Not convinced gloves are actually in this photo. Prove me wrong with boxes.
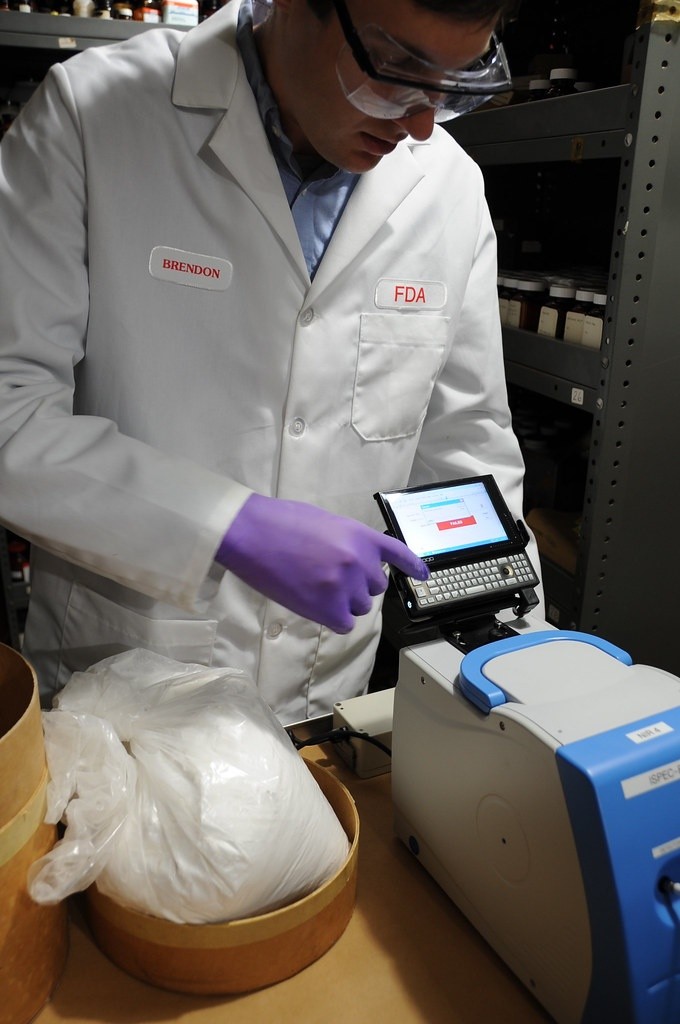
[213,491,429,635]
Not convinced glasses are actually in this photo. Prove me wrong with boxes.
[334,0,513,124]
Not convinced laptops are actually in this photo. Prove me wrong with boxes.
[374,474,539,617]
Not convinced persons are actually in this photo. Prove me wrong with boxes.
[1,0,548,728]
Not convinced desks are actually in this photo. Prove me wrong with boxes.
[34,739,554,1024]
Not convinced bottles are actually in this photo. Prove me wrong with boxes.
[545,69,578,99]
[498,267,608,350]
[523,80,550,104]
[513,407,570,516]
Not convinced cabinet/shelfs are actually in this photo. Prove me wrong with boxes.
[0,0,680,674]
